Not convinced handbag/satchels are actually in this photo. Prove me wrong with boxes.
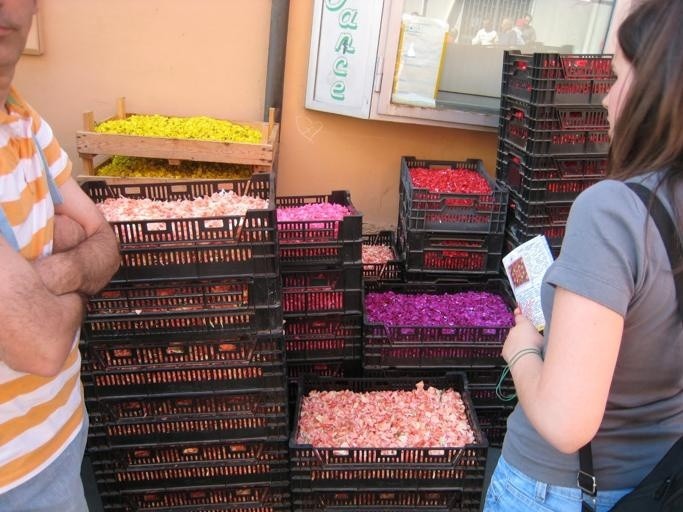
[547,181,682,511]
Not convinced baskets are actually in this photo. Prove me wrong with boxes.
[298,483,481,508]
[274,190,402,385]
[290,374,486,487]
[497,50,619,285]
[468,388,521,450]
[79,173,294,507]
[393,154,511,277]
[365,279,520,370]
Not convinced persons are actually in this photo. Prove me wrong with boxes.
[482,1,683,512]
[447,12,538,50]
[0,1,122,511]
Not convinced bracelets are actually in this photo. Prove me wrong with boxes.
[495,349,542,401]
